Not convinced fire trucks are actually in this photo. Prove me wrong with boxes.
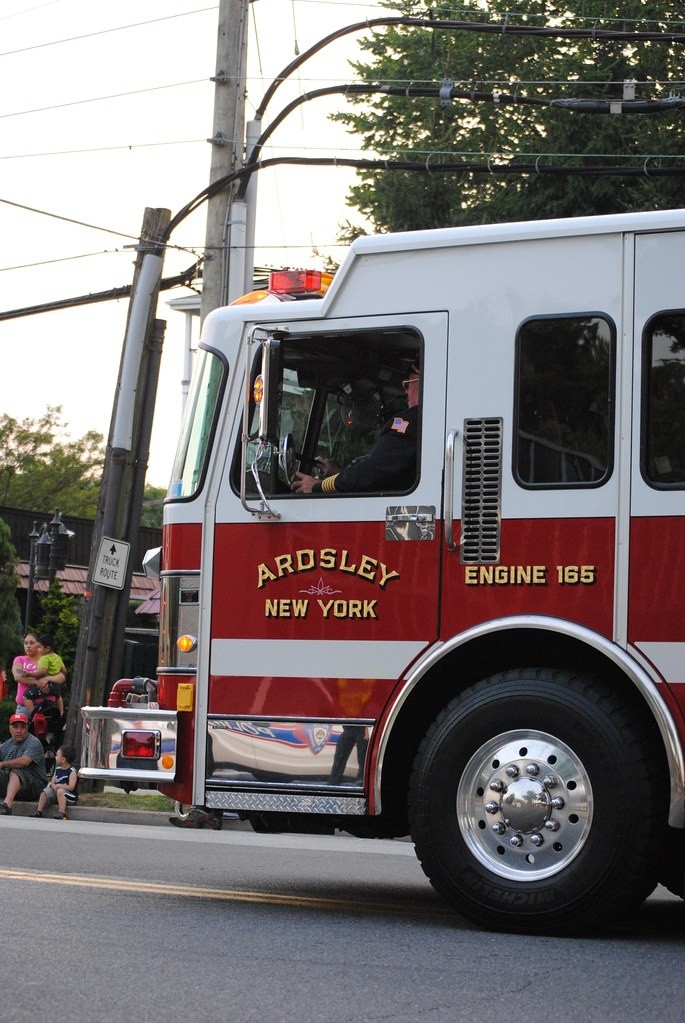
[75,210,685,938]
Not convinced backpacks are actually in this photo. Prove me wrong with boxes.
[28,691,61,747]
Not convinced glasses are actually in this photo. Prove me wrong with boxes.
[402,378,419,390]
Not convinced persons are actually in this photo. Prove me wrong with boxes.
[291,352,419,494]
[29,744,78,819]
[325,725,368,787]
[12,633,67,730]
[0,713,46,815]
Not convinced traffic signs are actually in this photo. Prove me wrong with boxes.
[91,535,132,590]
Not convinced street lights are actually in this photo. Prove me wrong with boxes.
[23,504,73,637]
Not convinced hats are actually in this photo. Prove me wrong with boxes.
[10,713,28,724]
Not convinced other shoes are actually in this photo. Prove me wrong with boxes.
[54,812,67,819]
[29,810,42,818]
[0,802,12,815]
